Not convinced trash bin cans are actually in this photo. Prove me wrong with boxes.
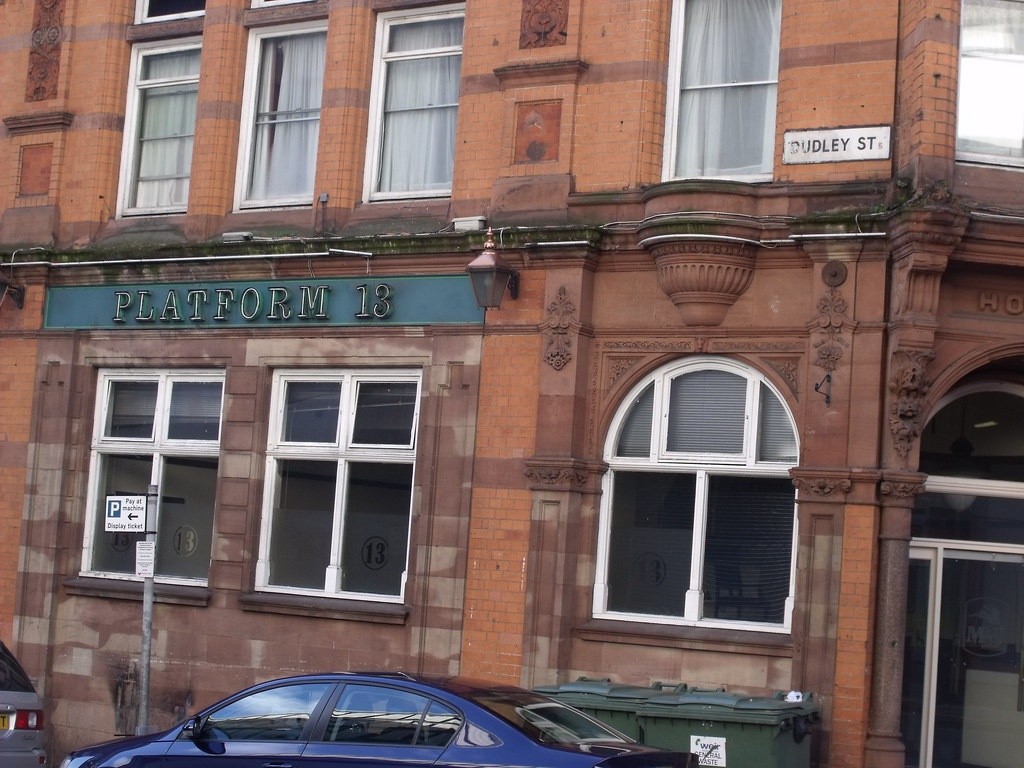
[636,685,823,768]
[530,676,688,744]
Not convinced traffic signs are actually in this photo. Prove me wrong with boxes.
[105,494,148,535]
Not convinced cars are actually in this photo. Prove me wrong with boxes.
[60,670,698,768]
[0,641,53,768]
